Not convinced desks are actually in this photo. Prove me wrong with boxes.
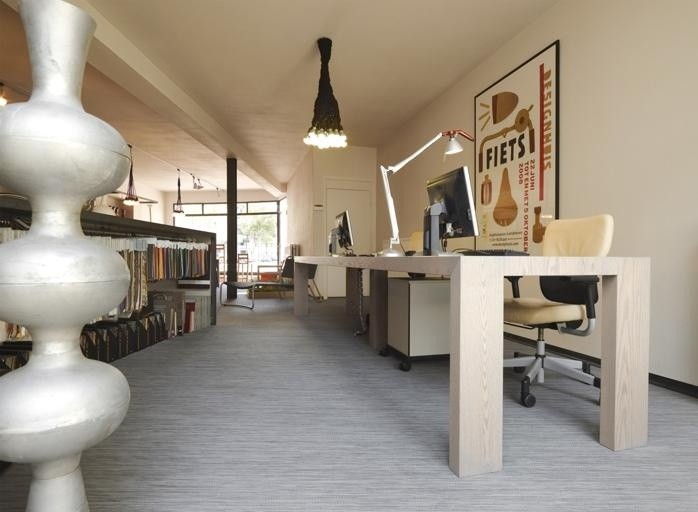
[290,252,652,478]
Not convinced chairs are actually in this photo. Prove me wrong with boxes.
[218,252,325,309]
[501,216,616,407]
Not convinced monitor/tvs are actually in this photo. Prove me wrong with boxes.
[424,165,478,256]
[329,209,353,257]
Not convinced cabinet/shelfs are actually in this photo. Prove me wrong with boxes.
[381,276,450,370]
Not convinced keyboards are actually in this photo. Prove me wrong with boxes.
[462,249,530,256]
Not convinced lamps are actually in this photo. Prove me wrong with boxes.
[123,141,223,217]
[302,38,347,151]
[379,131,476,259]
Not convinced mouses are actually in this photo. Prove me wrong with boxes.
[452,248,472,254]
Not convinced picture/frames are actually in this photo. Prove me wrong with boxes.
[471,40,560,256]
